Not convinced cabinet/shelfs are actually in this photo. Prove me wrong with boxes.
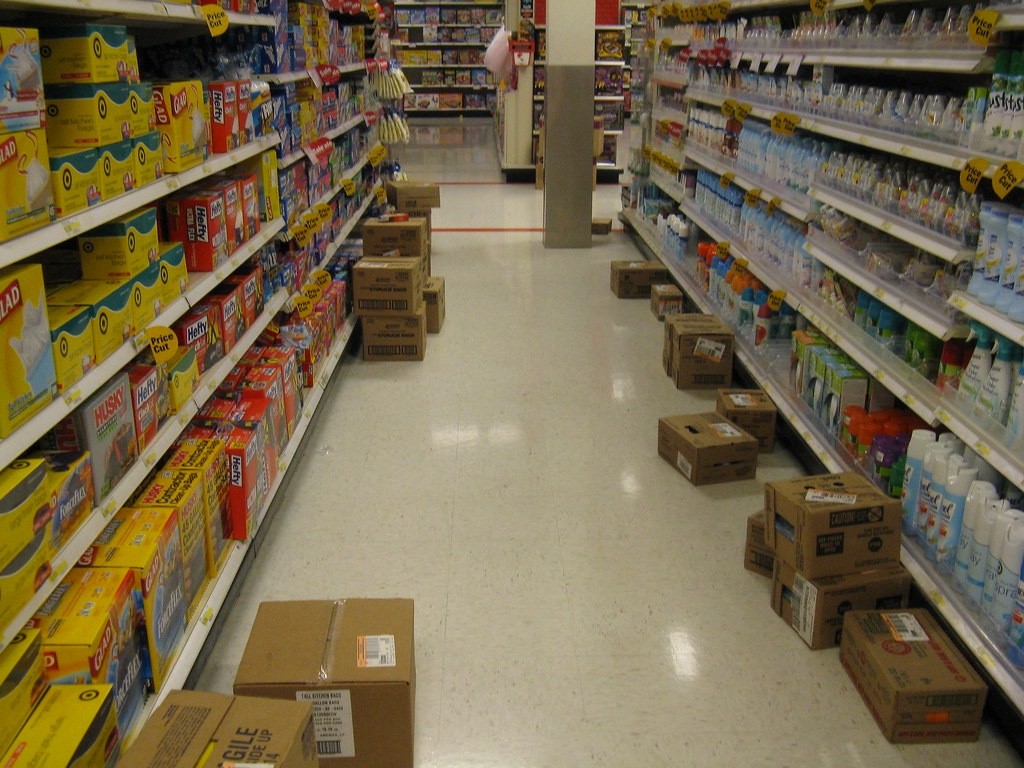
[531,24,626,171]
[272,61,392,393]
[391,0,504,112]
[620,13,1024,719]
[620,1,647,113]
[0,0,324,768]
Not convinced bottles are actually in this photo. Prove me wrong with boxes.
[695,171,825,357]
[656,207,698,257]
[853,290,934,377]
[899,429,1024,668]
[840,405,951,499]
[739,121,833,195]
[967,202,1024,323]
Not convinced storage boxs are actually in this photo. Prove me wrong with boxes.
[650,284,684,323]
[534,104,545,130]
[657,411,759,486]
[762,471,902,581]
[538,113,604,166]
[595,66,623,97]
[621,169,697,223]
[591,217,612,235]
[534,67,544,95]
[0,0,503,768]
[595,102,623,130]
[533,138,539,164]
[597,31,625,59]
[770,555,913,651]
[743,507,775,581]
[597,136,616,164]
[787,324,895,453]
[838,607,988,746]
[535,158,597,192]
[670,320,735,391]
[662,311,725,378]
[538,32,546,61]
[610,259,669,299]
[716,387,777,455]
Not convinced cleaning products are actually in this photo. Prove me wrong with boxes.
[638,1,1024,681]
